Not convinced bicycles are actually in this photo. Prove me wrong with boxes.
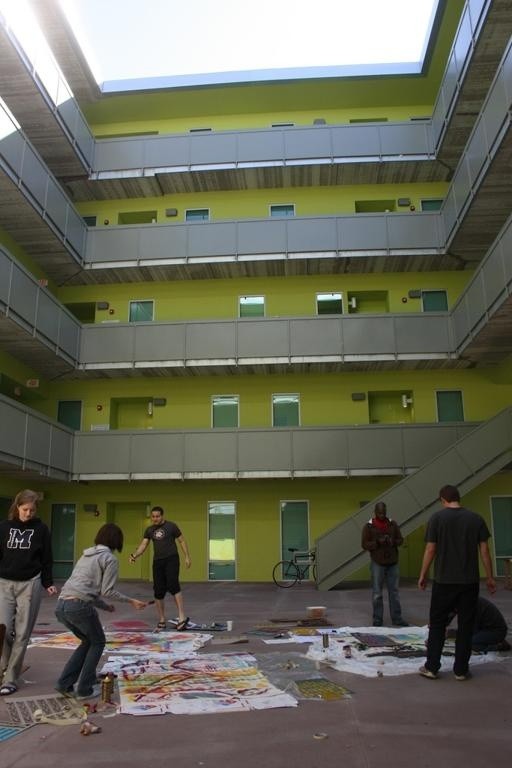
[273,546,321,587]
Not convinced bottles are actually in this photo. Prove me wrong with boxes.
[102,677,112,703]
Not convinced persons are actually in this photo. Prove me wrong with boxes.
[129,506,190,632]
[54,522,150,701]
[447,595,512,652]
[0,489,58,697]
[418,485,498,680]
[361,502,410,627]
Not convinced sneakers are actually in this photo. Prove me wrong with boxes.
[496,639,511,651]
[418,666,438,680]
[452,670,467,680]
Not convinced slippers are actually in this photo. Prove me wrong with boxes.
[0,687,17,696]
[55,686,76,698]
[157,621,166,628]
[78,688,101,700]
[176,617,190,632]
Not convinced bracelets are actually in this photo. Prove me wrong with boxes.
[130,553,136,560]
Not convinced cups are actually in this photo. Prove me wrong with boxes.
[343,646,351,658]
[226,621,232,632]
[323,633,329,647]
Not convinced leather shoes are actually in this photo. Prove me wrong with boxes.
[392,618,409,627]
[373,619,384,627]
